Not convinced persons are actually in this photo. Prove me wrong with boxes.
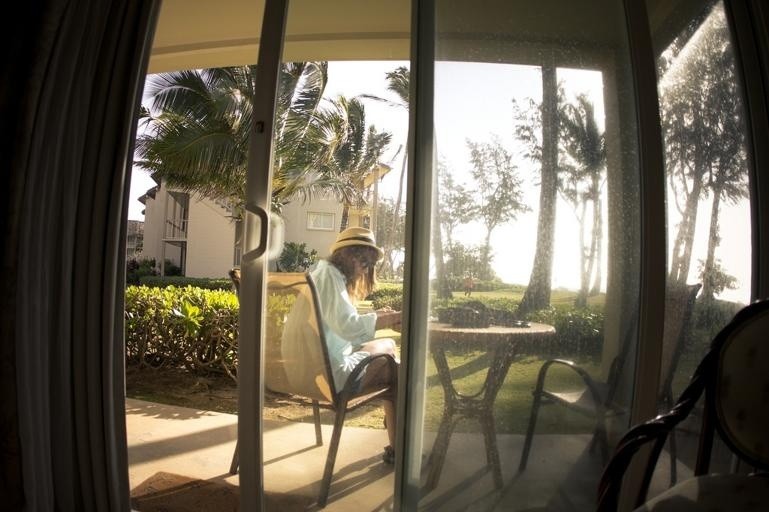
[281,225,429,463]
[465,274,476,297]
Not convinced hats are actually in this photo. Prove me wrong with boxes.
[329,227,384,261]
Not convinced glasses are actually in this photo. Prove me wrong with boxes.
[350,248,369,268]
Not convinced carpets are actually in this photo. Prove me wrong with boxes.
[129,466,312,511]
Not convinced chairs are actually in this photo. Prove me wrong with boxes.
[521,277,704,491]
[594,299,768,512]
[224,267,399,509]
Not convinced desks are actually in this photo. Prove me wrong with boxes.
[408,313,560,492]
